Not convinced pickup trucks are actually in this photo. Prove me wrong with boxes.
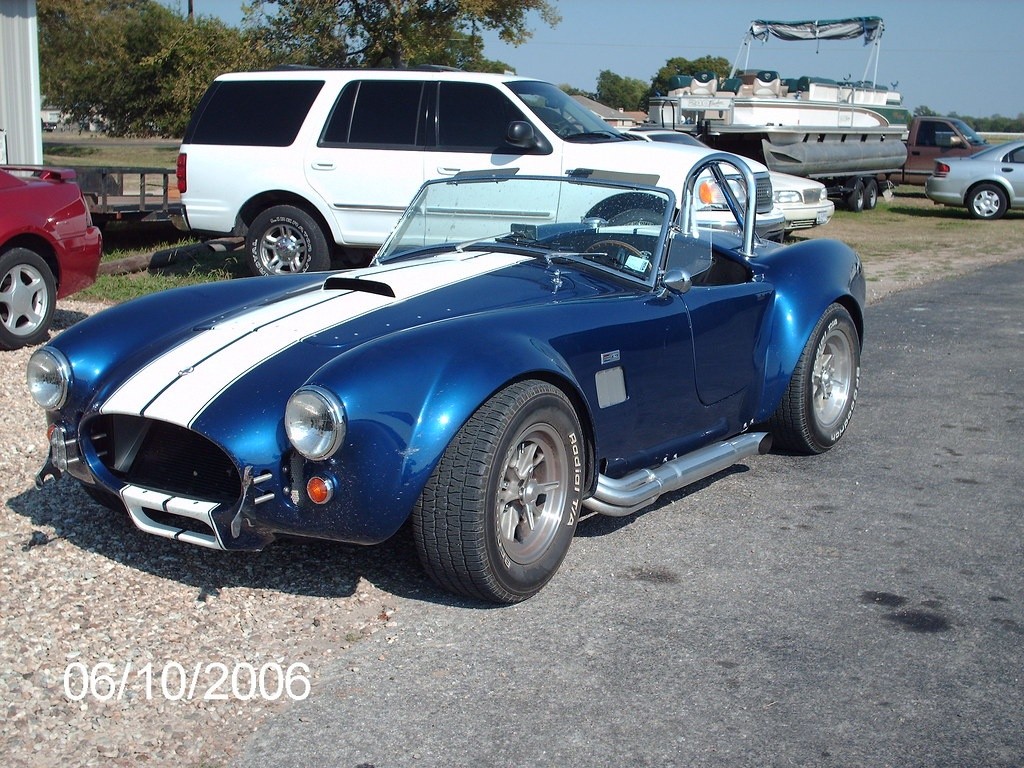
[835,115,998,195]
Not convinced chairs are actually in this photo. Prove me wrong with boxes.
[666,71,889,123]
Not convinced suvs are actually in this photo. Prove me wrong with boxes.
[175,62,787,279]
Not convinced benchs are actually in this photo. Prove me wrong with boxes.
[551,235,746,286]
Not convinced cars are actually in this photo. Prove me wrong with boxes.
[610,129,835,232]
[924,140,1024,220]
[0,166,105,353]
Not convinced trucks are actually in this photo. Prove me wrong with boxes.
[628,68,908,213]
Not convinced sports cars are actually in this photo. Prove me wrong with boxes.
[27,152,868,605]
